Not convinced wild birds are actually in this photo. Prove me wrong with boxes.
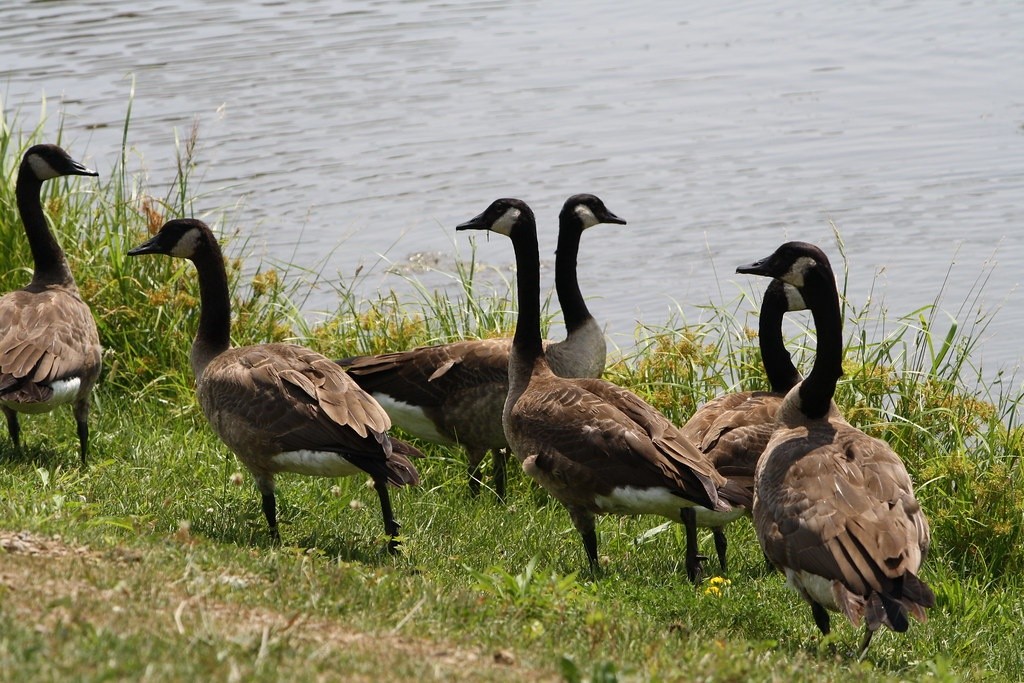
[337,194,629,503]
[127,217,422,564]
[675,275,808,573]
[0,143,104,465]
[455,197,746,586]
[733,240,938,653]
